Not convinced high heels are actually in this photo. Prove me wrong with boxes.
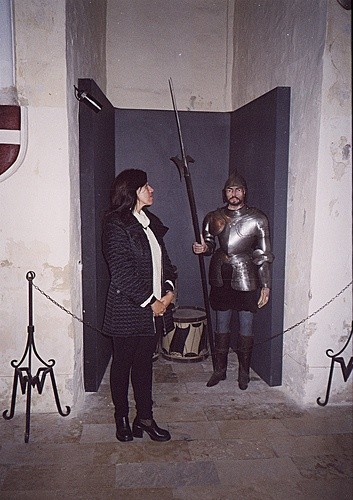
[114,413,134,442]
[133,416,171,441]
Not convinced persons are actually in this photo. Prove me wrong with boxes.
[101,168,178,442]
[191,172,274,390]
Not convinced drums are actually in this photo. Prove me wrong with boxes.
[161,305,210,363]
